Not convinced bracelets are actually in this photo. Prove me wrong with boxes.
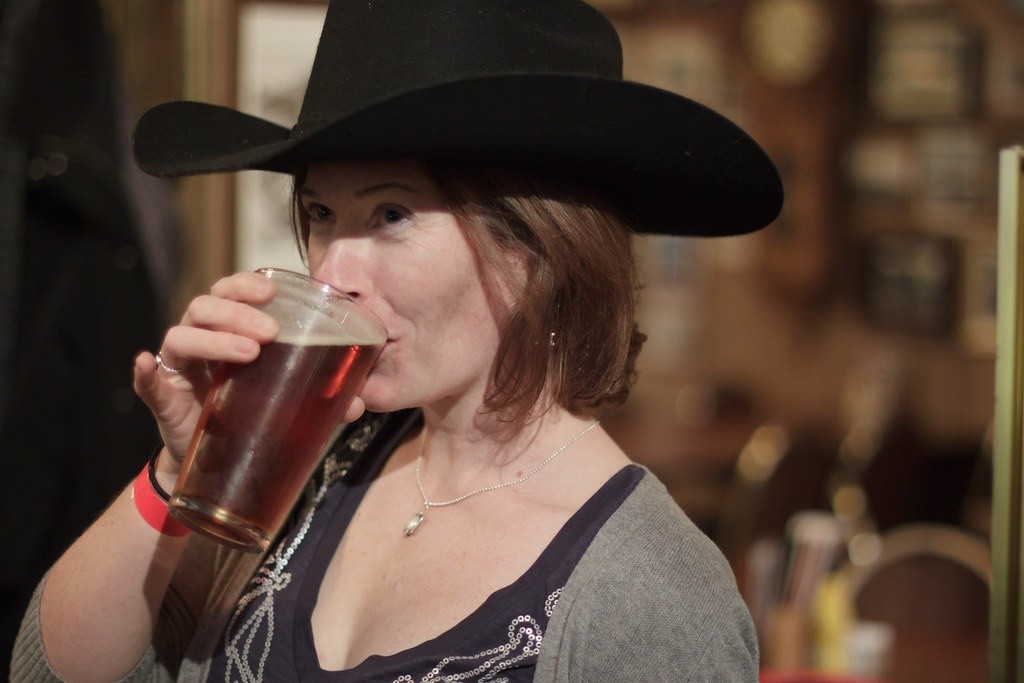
[134,444,192,537]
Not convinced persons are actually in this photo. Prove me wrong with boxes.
[10,0,786,683]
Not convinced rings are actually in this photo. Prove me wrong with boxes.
[156,351,191,374]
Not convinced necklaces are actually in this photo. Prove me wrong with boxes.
[404,420,601,535]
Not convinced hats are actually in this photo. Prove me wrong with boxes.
[130,0,786,238]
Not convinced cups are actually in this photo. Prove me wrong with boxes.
[169,268,388,551]
